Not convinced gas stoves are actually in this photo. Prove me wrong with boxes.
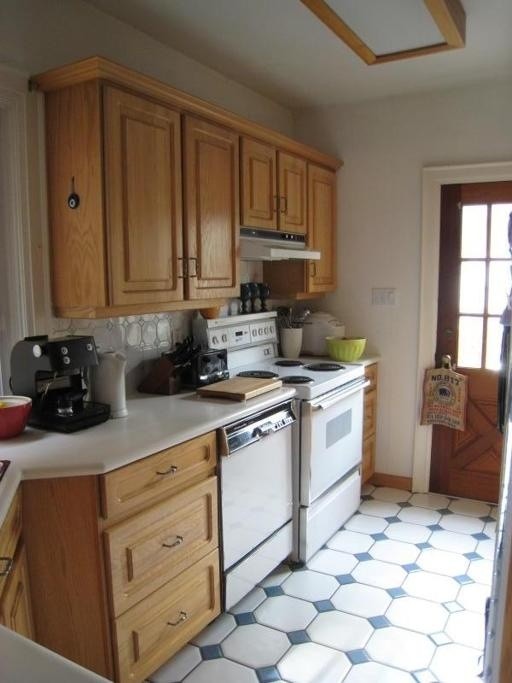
[227,355,366,400]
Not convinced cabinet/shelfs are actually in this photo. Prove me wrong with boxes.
[360,363,379,490]
[34,53,240,322]
[0,480,38,644]
[22,432,220,683]
[237,112,308,241]
[263,139,348,305]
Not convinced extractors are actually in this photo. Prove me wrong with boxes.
[238,225,323,264]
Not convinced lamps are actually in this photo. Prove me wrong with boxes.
[296,0,469,69]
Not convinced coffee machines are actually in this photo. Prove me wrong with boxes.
[10,330,116,432]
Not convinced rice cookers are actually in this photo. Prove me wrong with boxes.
[298,311,347,358]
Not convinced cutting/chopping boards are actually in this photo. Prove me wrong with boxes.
[194,373,283,400]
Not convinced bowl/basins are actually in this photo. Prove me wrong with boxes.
[1,394,33,440]
[200,305,218,318]
[322,334,367,362]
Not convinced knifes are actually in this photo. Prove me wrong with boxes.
[167,330,203,367]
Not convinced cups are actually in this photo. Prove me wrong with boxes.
[279,328,303,360]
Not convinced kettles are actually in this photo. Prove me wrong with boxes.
[89,347,132,419]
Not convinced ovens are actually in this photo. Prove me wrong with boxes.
[298,378,371,564]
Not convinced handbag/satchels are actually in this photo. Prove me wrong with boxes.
[420,361,467,432]
